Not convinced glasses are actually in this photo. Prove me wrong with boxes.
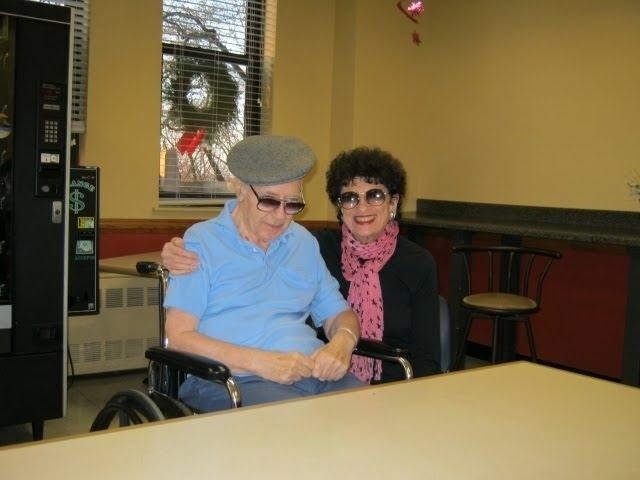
[250,184,306,213]
[337,189,392,210]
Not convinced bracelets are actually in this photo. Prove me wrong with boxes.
[336,326,358,343]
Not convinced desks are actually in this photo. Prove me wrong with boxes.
[2,354,640,478]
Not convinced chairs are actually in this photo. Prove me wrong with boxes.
[448,243,562,368]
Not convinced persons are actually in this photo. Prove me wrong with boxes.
[162,134,369,411]
[160,145,439,381]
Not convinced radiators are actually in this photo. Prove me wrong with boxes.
[63,272,162,377]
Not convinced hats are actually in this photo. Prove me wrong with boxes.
[227,134,316,185]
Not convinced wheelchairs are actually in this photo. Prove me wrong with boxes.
[87,260,416,434]
